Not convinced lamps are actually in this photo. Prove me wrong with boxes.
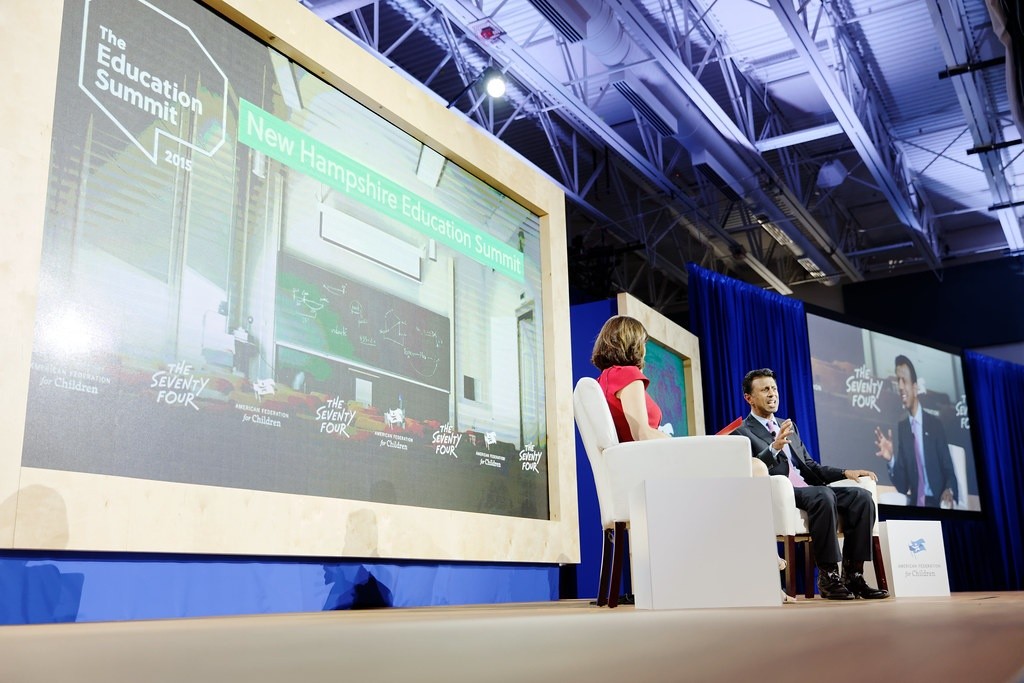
[446,66,506,110]
[816,157,849,190]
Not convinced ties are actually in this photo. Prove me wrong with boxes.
[910,420,926,506]
[767,421,809,487]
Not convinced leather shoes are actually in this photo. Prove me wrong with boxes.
[841,573,890,599]
[817,572,855,599]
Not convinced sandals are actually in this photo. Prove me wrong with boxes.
[782,595,796,603]
[779,559,787,570]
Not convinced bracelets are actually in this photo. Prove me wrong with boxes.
[841,468,847,480]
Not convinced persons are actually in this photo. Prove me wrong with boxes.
[593,314,670,442]
[728,369,892,602]
[874,355,959,510]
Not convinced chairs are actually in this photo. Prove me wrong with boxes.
[572,377,890,609]
